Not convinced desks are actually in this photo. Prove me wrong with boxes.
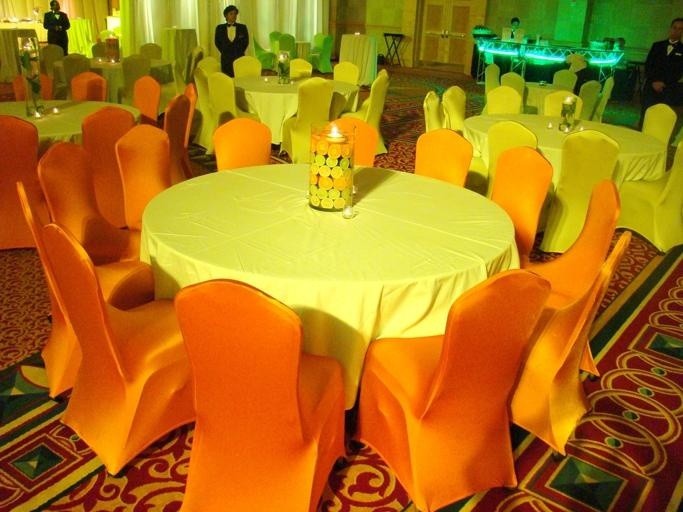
[139,163,521,410]
[67,19,92,58]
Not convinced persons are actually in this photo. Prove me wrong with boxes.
[501,17,525,43]
[638,18,683,130]
[44,0,70,57]
[214,5,248,78]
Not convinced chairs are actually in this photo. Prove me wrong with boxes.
[173,278,348,512]
[413,127,473,187]
[422,29,683,255]
[42,223,195,477]
[520,179,622,293]
[0,29,391,253]
[507,229,634,457]
[11,181,154,401]
[489,146,553,261]
[36,141,142,266]
[352,268,552,511]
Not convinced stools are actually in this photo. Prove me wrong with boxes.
[381,32,405,66]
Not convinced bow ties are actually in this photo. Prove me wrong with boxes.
[228,23,236,27]
[667,42,675,47]
